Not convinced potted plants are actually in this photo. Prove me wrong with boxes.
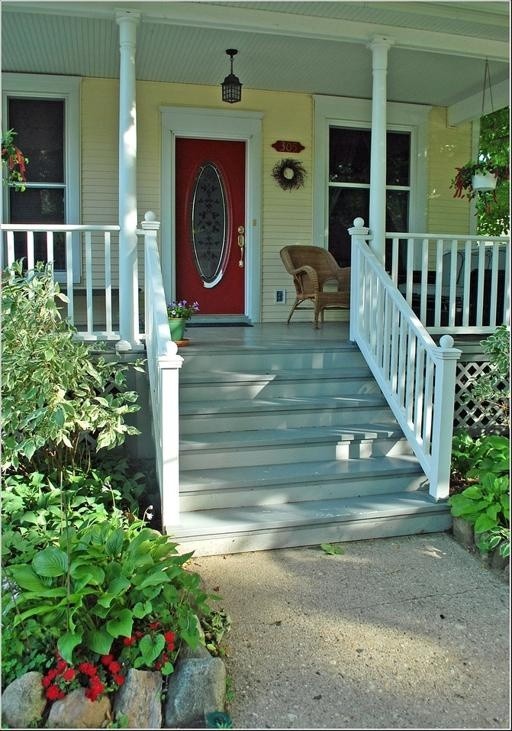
[448,158,509,208]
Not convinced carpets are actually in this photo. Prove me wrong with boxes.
[185,324,253,327]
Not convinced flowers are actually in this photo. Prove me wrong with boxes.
[167,300,201,321]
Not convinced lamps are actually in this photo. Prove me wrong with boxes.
[220,49,243,104]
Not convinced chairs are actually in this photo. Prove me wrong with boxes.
[279,245,351,330]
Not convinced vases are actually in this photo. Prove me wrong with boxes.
[168,318,186,341]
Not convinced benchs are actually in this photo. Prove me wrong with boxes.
[398,246,505,313]
